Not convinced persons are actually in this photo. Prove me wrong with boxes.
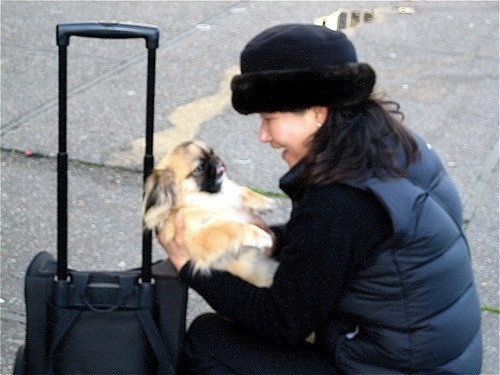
[157,24,482,375]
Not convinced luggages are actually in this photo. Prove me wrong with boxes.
[7,21,192,374]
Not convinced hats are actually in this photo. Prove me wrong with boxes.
[231,23,375,115]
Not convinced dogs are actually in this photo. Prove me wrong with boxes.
[142,140,293,288]
